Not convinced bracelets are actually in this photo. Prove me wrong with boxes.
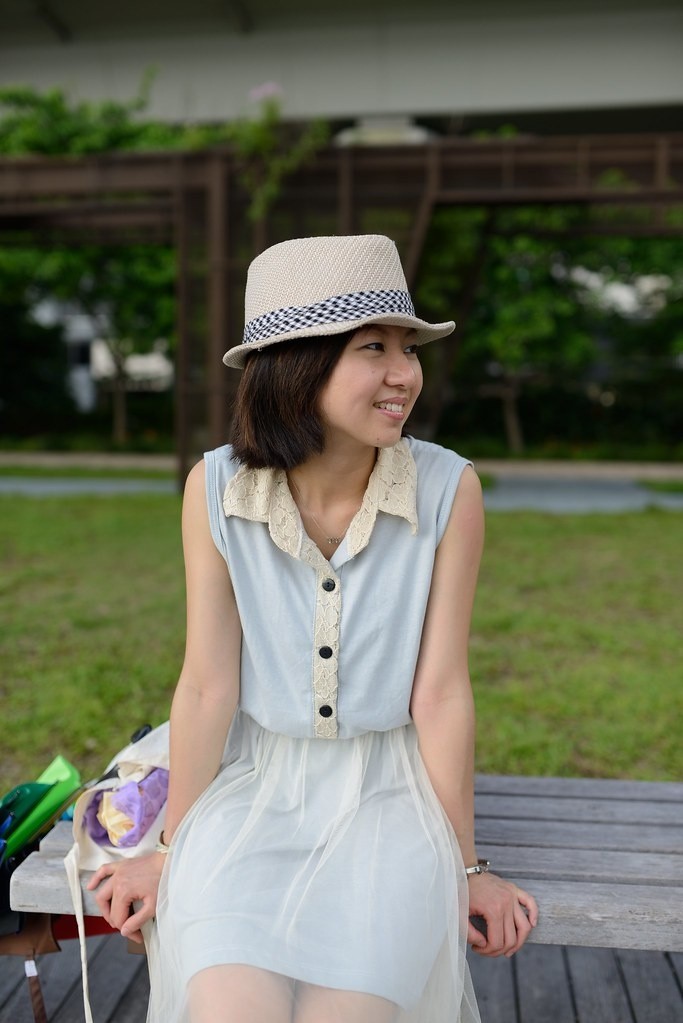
[465,859,490,874]
[156,829,169,853]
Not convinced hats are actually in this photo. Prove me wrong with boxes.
[222,234,456,370]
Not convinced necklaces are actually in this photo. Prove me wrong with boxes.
[288,470,362,544]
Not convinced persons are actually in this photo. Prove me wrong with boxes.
[87,234,539,1023]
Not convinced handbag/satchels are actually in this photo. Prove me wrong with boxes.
[72,719,170,872]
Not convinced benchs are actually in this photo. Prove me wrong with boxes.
[10,771,683,962]
